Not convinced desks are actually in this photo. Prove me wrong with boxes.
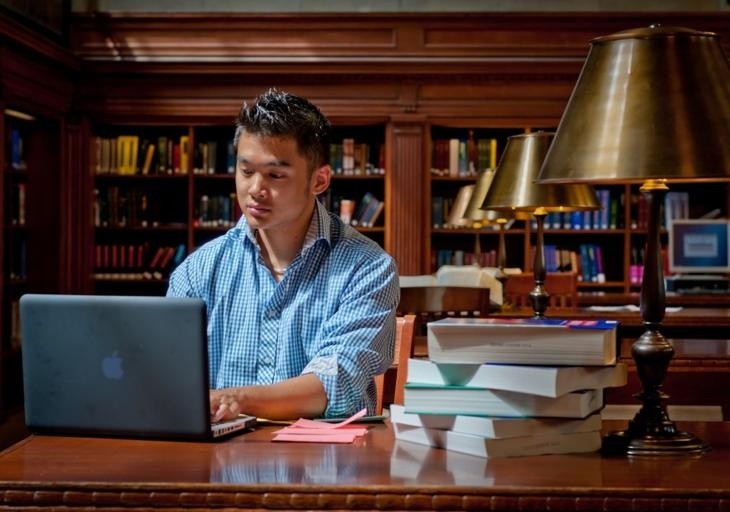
[0,424,730,511]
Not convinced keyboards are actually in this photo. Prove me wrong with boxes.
[678,288,729,295]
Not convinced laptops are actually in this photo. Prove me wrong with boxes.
[19,293,256,440]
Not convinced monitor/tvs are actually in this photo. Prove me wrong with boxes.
[668,219,730,273]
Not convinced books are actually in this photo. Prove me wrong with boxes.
[541,242,606,282]
[318,135,385,232]
[659,191,690,234]
[390,316,629,456]
[630,245,645,284]
[430,181,515,230]
[94,134,243,281]
[429,131,517,178]
[531,188,652,231]
[431,249,497,273]
[7,124,33,351]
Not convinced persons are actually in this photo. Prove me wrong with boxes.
[168,85,402,424]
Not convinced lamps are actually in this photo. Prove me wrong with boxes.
[533,23,730,460]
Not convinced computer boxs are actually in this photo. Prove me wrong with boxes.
[665,276,729,291]
